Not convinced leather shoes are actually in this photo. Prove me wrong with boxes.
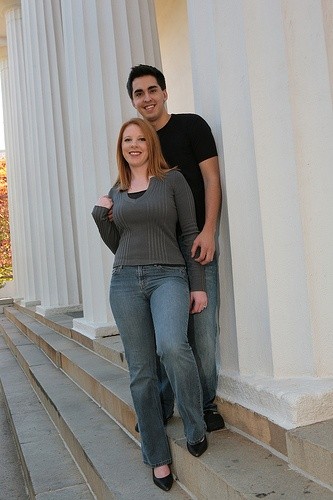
[187,434,208,457]
[152,467,173,491]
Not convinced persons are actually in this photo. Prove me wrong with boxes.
[126,64,227,434]
[91,117,210,493]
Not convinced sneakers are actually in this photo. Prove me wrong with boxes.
[135,411,174,433]
[203,410,225,432]
